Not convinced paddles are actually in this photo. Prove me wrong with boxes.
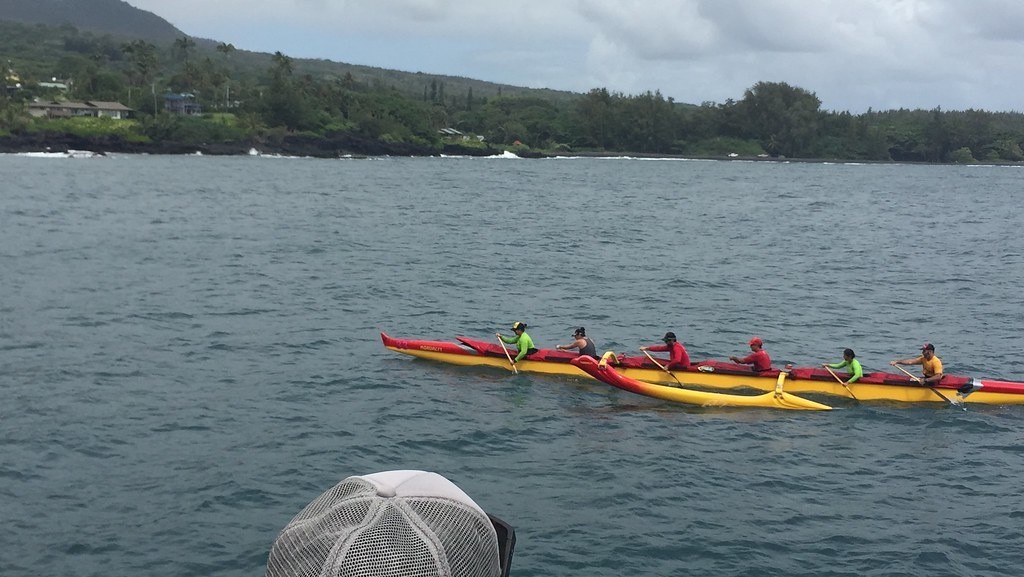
[893,363,952,404]
[641,348,686,389]
[823,365,857,401]
[495,335,519,376]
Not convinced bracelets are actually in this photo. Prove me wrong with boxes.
[514,359,517,363]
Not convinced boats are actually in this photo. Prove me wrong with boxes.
[379,332,1024,411]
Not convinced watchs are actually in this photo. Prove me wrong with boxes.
[924,378,927,383]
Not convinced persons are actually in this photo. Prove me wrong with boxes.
[729,337,772,371]
[556,327,599,360]
[889,343,943,385]
[822,348,862,386]
[265,470,501,577]
[496,322,538,366]
[640,332,690,371]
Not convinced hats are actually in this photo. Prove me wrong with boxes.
[571,329,585,336]
[511,322,524,329]
[660,331,675,340]
[747,337,762,345]
[918,343,934,350]
[262,469,517,577]
